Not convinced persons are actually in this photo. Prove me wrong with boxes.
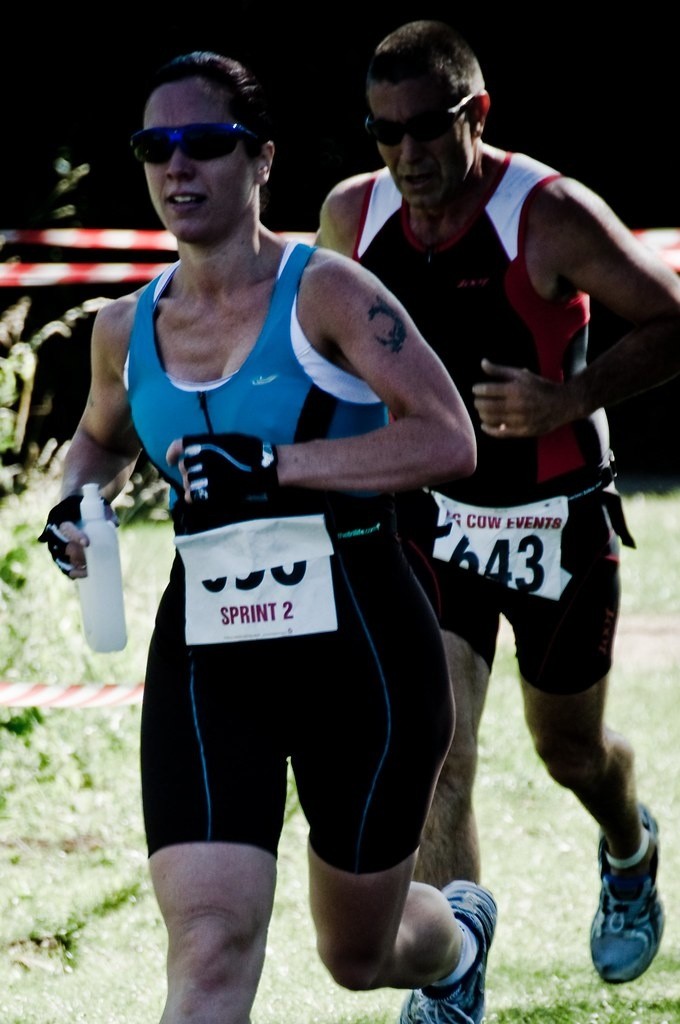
[41,49,498,1024]
[312,19,680,986]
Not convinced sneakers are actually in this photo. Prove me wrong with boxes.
[400,879,497,1024]
[590,800,665,984]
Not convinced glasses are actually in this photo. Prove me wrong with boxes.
[364,90,479,147]
[127,122,263,165]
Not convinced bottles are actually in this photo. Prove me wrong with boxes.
[76,484,127,652]
